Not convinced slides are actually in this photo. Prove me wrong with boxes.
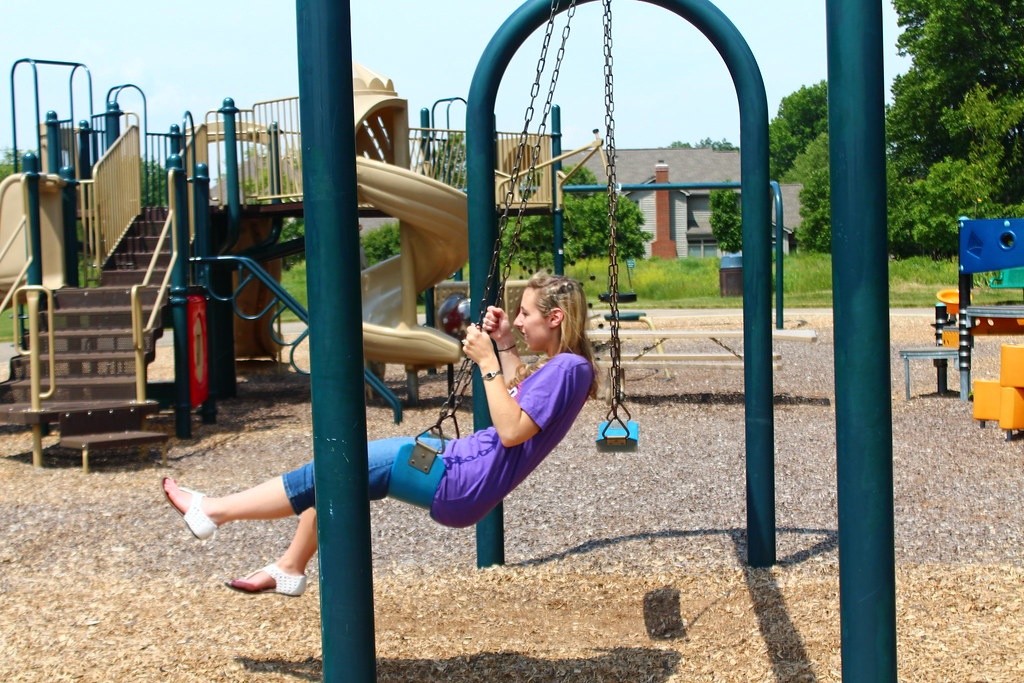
[355,77,476,369]
[180,122,283,364]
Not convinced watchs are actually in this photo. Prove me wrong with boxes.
[481,370,502,381]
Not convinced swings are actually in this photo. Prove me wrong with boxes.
[381,0,577,510]
[593,1,642,454]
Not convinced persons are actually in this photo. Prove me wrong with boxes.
[161,273,602,598]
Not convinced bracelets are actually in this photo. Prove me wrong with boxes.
[498,345,516,352]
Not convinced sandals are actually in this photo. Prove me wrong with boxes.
[224,563,307,597]
[160,476,218,540]
